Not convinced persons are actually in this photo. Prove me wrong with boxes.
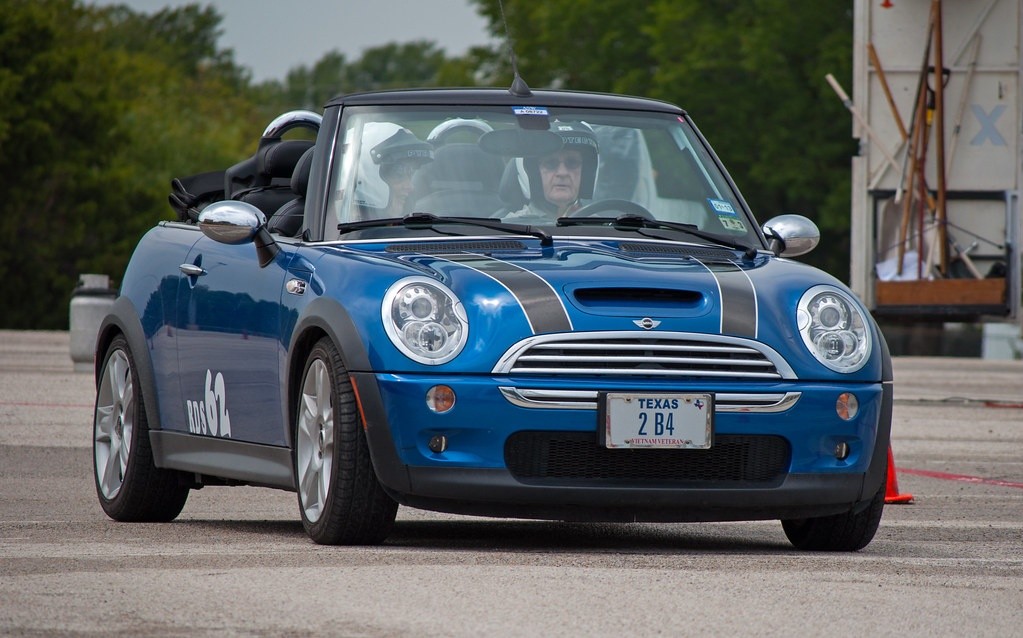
[501,115,604,221]
[322,120,436,242]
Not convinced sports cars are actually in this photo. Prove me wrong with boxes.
[93,77,894,551]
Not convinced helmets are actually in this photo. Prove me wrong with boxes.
[515,118,600,200]
[427,117,496,142]
[333,121,434,223]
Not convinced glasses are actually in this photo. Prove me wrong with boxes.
[389,162,419,179]
[542,157,583,169]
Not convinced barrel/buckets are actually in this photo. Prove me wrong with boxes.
[980,322,1023,361]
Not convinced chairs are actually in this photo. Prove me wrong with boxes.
[224,110,532,236]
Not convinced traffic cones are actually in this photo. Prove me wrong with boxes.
[884,439,914,503]
